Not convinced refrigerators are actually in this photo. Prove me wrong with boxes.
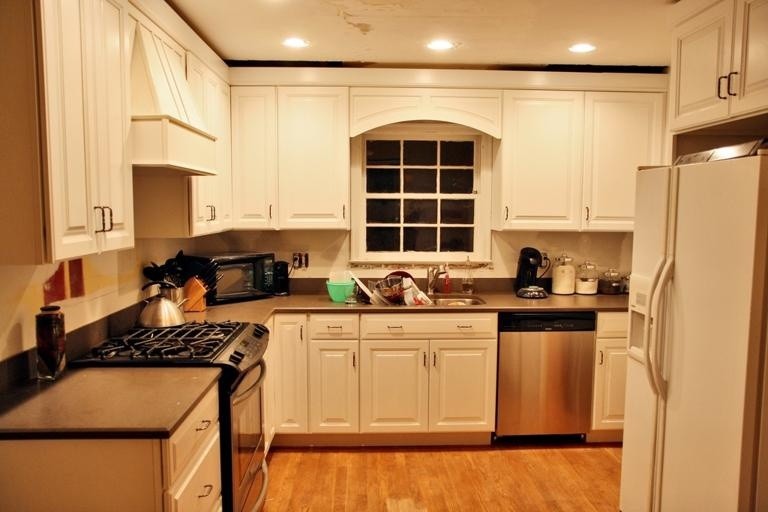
[613,152,768,512]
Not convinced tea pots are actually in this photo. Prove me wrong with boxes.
[135,278,191,329]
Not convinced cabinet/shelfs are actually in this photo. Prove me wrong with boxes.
[586,311,630,445]
[0,379,224,512]
[229,67,351,232]
[308,311,359,446]
[669,0,768,137]
[359,312,498,447]
[260,312,276,459]
[274,313,308,447]
[490,92,671,232]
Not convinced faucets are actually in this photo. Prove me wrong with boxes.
[427,265,445,295]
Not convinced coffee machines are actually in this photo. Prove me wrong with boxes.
[513,246,548,298]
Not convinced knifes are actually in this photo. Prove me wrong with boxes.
[195,257,225,291]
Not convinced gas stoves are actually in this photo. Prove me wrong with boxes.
[72,318,271,372]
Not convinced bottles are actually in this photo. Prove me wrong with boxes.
[575,258,599,296]
[548,253,575,295]
[442,275,452,293]
[461,255,474,295]
[598,261,625,295]
[32,304,68,383]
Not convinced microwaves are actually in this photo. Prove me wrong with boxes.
[175,248,276,305]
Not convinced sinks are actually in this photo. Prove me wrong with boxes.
[436,299,485,305]
[360,298,436,305]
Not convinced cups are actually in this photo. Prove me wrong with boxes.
[158,286,185,312]
[325,279,357,303]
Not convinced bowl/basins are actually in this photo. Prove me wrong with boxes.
[375,276,404,304]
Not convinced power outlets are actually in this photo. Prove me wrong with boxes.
[540,253,547,268]
[293,252,303,270]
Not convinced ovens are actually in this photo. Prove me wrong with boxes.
[217,360,269,512]
